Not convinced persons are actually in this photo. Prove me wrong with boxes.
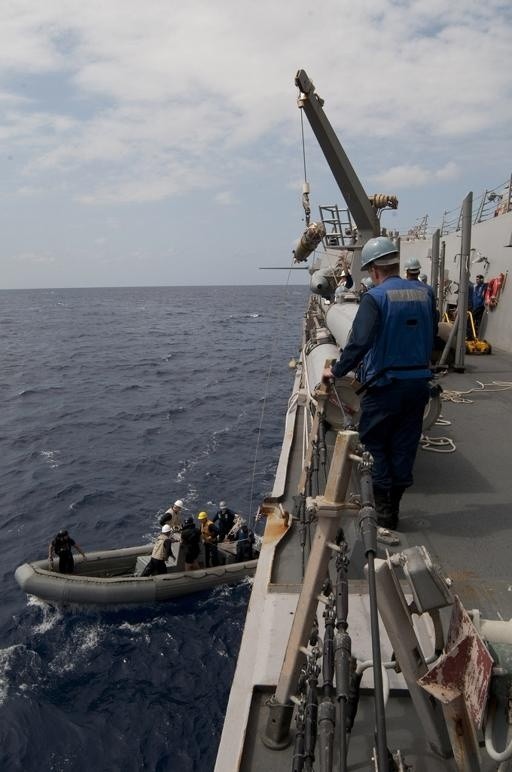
[150,497,239,578]
[321,235,441,533]
[46,529,89,576]
[334,256,491,342]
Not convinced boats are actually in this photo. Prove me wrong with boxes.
[16,533,270,614]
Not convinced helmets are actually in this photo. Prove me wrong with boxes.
[404,258,421,271]
[218,501,228,509]
[161,524,172,533]
[183,517,194,524]
[58,529,66,535]
[360,237,400,271]
[419,274,428,281]
[174,500,183,508]
[198,512,209,520]
